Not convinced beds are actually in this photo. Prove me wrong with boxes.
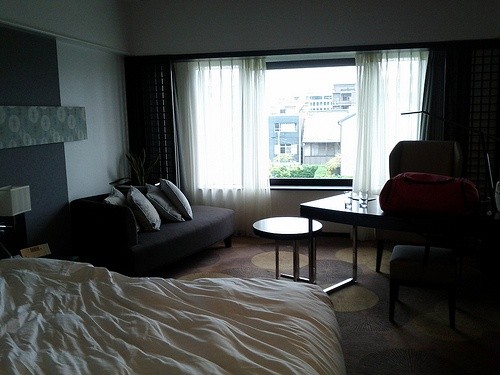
[0,258,346,375]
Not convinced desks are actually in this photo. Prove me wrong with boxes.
[299,192,384,283]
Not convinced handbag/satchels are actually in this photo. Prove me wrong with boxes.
[380,172,480,216]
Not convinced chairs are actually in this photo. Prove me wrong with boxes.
[375,141,481,330]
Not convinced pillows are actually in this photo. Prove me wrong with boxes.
[126,185,161,231]
[160,178,194,220]
[103,187,140,232]
[145,183,185,222]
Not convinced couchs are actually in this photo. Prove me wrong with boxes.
[69,193,233,270]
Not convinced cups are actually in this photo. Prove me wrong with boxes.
[343,190,353,209]
[358,191,368,208]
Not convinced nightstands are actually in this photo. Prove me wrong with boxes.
[37,245,72,260]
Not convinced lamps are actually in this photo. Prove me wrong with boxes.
[0,185,32,255]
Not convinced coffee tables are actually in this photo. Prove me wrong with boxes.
[252,217,323,282]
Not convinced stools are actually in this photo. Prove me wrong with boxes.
[389,245,474,330]
